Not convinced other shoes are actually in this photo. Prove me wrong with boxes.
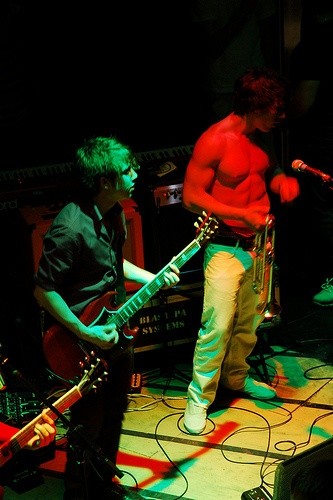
[314,278,333,303]
[106,484,143,500]
[244,377,277,400]
[183,400,207,434]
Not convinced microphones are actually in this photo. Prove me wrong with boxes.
[0,354,22,377]
[292,160,330,183]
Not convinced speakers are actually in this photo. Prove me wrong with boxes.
[137,173,205,285]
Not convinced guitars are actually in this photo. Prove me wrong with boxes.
[39,210,220,384]
[0,349,111,482]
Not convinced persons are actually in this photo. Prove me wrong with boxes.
[35,137,180,500]
[1,414,56,450]
[181,72,300,435]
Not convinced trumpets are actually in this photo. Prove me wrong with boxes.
[252,211,283,318]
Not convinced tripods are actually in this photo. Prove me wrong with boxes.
[140,290,193,382]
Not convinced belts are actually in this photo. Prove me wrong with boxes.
[208,232,256,249]
